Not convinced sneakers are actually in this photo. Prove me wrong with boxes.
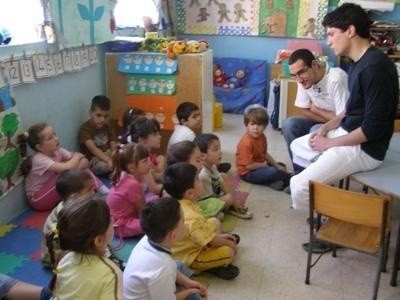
[207,263,239,280]
[303,217,342,254]
[265,180,284,190]
[229,205,252,219]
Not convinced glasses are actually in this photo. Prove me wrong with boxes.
[290,66,310,79]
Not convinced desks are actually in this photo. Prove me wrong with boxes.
[339,132,400,286]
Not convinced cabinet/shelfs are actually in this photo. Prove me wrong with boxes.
[105,50,213,155]
[337,27,400,132]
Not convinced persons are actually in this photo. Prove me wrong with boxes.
[290,3,399,252]
[236,104,290,190]
[282,49,349,173]
[1,95,249,299]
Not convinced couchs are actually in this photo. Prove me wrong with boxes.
[213,57,267,114]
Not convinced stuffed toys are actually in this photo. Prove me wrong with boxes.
[223,68,248,89]
[213,64,228,86]
[141,37,209,58]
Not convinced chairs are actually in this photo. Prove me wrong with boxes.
[305,179,393,300]
